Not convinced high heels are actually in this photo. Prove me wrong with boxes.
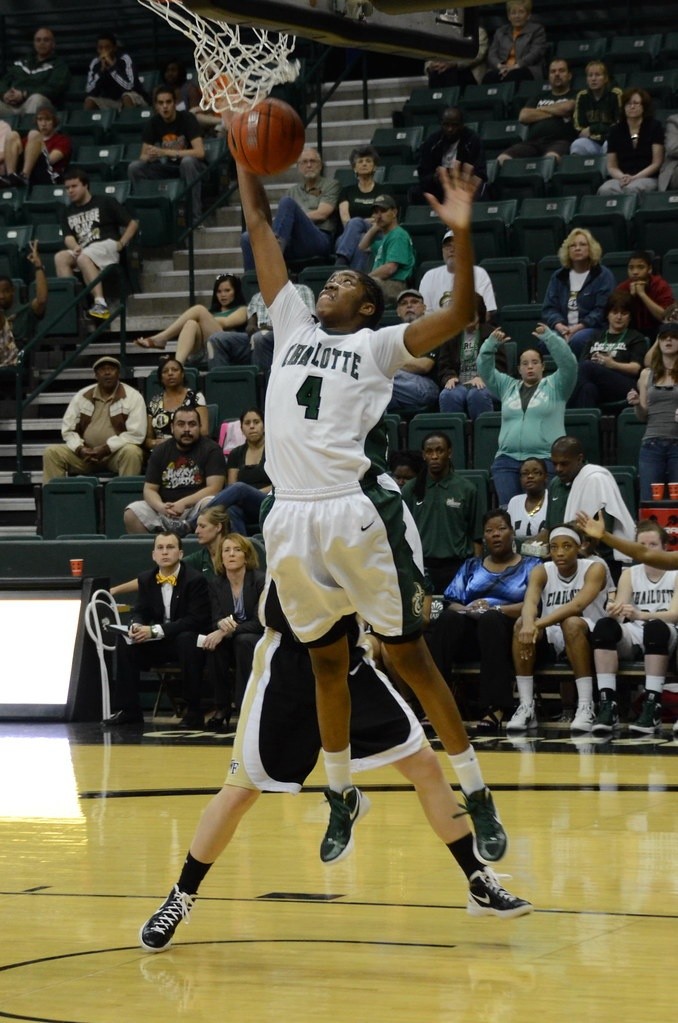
[476,705,503,730]
[209,705,232,723]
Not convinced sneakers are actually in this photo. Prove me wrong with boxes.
[466,864,533,917]
[82,303,110,320]
[629,690,663,734]
[570,700,596,731]
[140,953,202,1010]
[319,784,369,864]
[590,688,620,733]
[465,787,509,864]
[138,883,200,953]
[505,700,537,730]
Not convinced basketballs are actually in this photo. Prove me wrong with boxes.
[227,97,307,177]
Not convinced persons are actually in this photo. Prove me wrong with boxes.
[139,454,532,954]
[1,0,678,736]
[203,68,508,865]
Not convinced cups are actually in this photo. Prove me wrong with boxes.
[70,559,84,577]
[668,483,678,500]
[650,484,665,500]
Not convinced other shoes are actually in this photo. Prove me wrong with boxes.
[159,512,188,539]
[0,171,29,189]
[100,710,144,725]
[176,713,205,727]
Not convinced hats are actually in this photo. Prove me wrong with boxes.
[658,323,678,337]
[36,104,56,118]
[93,356,121,374]
[442,230,454,243]
[397,289,423,303]
[370,195,396,210]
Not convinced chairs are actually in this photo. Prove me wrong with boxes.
[0,0,677,694]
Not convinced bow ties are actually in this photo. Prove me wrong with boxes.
[156,573,177,586]
